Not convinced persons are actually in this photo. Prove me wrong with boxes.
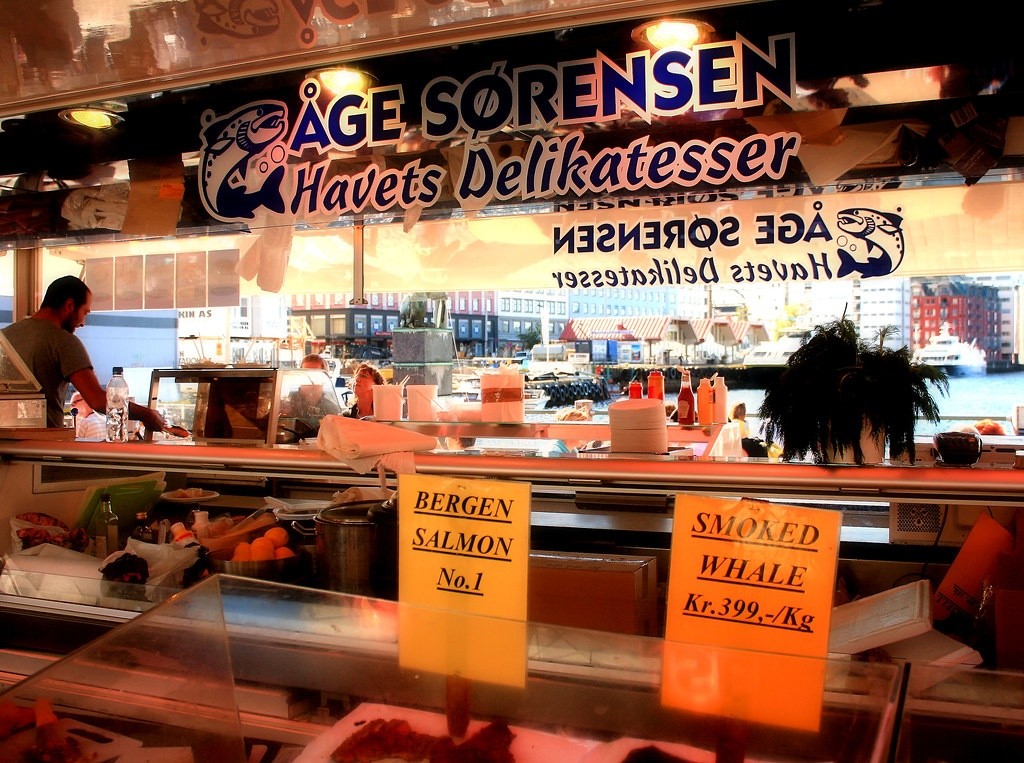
[67,386,110,438]
[0,275,171,435]
[279,355,386,420]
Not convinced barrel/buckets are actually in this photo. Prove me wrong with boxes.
[371,384,404,421]
[405,384,438,421]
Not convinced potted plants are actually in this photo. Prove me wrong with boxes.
[754,301,948,468]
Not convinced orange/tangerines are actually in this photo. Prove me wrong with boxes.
[231,527,295,561]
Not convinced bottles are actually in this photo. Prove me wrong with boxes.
[711,377,728,425]
[628,380,643,400]
[697,379,714,426]
[132,511,152,544]
[95,494,119,560]
[169,521,214,577]
[646,369,665,401]
[191,511,211,540]
[677,371,695,425]
[106,366,129,444]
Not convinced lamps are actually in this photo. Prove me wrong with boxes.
[58,102,124,131]
[303,62,380,92]
[630,14,716,48]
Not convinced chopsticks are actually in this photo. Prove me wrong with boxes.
[399,375,410,386]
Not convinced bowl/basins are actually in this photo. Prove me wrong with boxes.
[212,554,298,584]
[932,431,983,465]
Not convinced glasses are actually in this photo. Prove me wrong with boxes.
[354,374,372,380]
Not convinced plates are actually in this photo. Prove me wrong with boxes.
[583,735,762,763]
[160,491,220,503]
[271,503,328,537]
[288,701,603,763]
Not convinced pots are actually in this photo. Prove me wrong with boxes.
[313,499,398,601]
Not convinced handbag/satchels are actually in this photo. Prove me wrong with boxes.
[233,212,295,293]
